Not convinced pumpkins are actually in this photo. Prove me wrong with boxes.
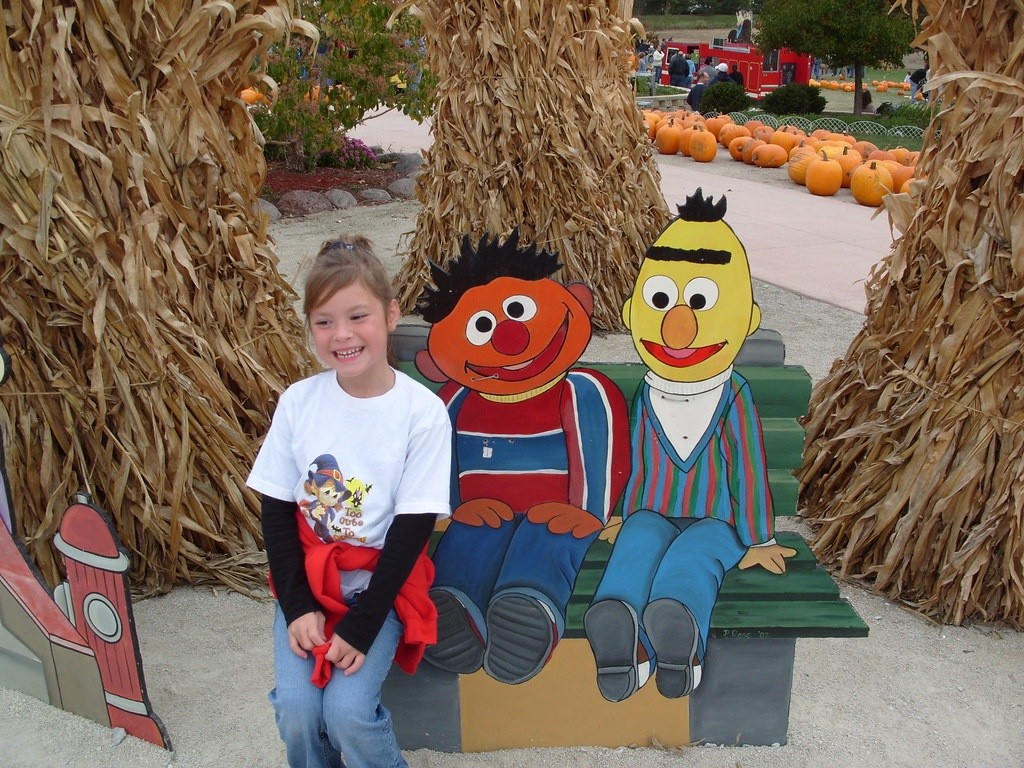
[241,84,329,107]
[640,110,927,207]
[810,79,911,95]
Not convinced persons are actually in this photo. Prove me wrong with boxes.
[242,233,453,768]
[813,57,865,80]
[635,37,746,112]
[904,64,945,103]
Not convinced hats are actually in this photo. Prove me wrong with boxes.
[715,63,728,72]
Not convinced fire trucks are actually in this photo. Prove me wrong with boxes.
[657,36,812,102]
